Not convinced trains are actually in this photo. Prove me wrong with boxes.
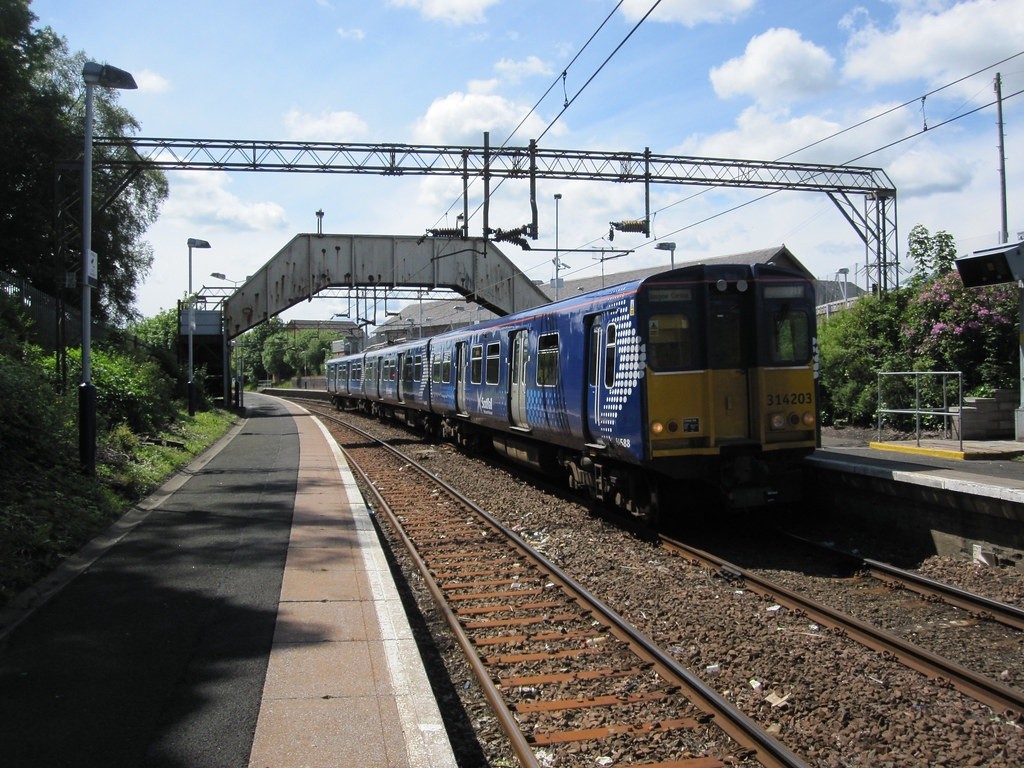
[323,264,816,518]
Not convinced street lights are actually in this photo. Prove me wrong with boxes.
[453,305,484,326]
[554,194,562,301]
[654,242,676,270]
[301,351,307,389]
[210,273,252,407]
[78,62,138,475]
[187,238,211,416]
[405,317,432,339]
[836,268,849,300]
